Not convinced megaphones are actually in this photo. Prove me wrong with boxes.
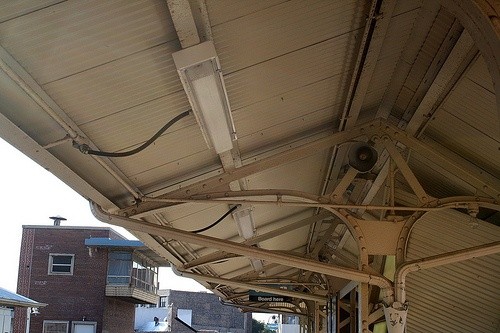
[346,142,379,174]
